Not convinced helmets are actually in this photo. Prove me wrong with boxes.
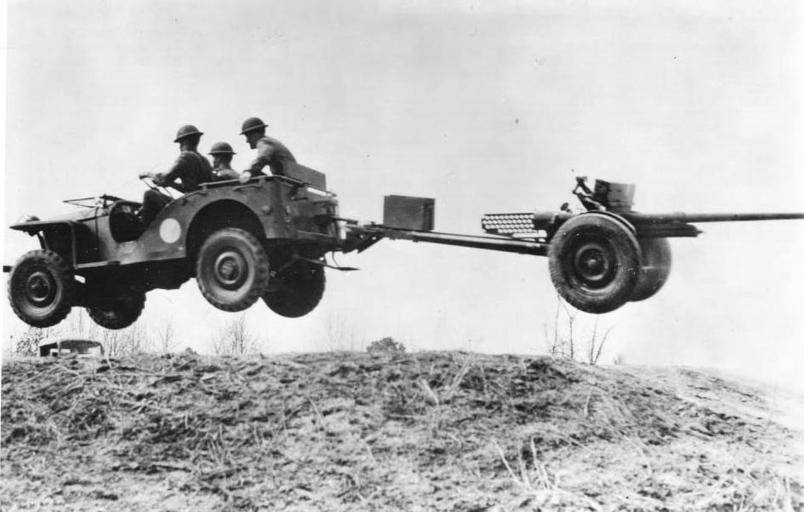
[174,125,203,142]
[209,142,237,156]
[240,117,269,135]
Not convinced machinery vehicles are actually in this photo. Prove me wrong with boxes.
[2,173,355,330]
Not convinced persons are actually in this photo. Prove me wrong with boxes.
[239,114,297,185]
[125,125,212,243]
[206,142,241,179]
